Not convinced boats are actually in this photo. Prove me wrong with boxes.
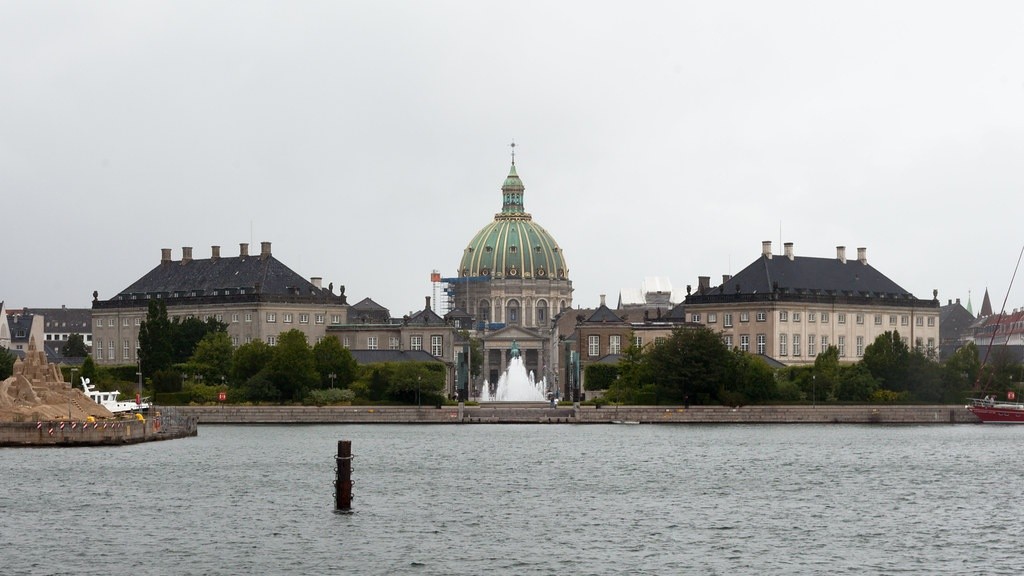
[80,377,151,414]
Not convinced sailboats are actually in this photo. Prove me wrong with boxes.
[965,246,1024,424]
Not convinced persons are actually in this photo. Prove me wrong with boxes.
[985,394,995,403]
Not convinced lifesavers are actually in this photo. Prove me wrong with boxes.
[134,393,141,404]
[1007,391,1015,400]
[154,418,161,430]
[218,392,227,401]
